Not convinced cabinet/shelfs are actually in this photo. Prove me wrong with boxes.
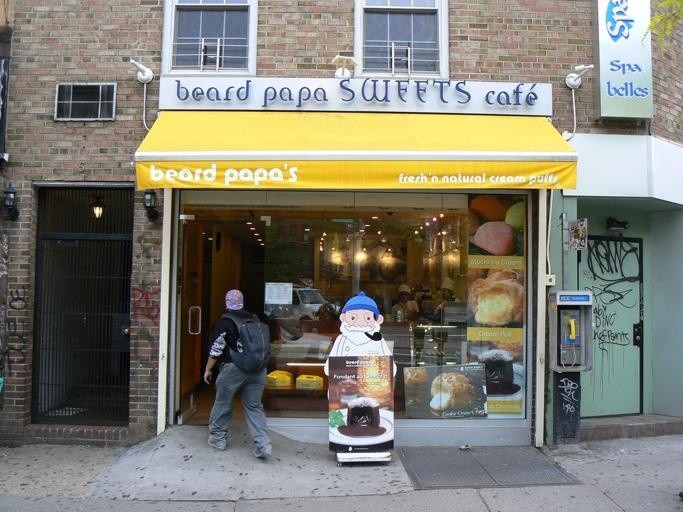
[383,326,465,366]
[264,321,339,411]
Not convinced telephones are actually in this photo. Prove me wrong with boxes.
[568,319,576,340]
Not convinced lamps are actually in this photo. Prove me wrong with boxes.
[3,181,19,221]
[561,61,595,143]
[604,216,630,231]
[139,187,159,222]
[88,190,106,222]
[128,57,154,133]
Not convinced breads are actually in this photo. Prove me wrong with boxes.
[469,195,526,256]
[468,268,523,326]
[404,367,427,385]
[430,372,474,412]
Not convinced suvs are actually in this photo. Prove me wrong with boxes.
[264,287,340,331]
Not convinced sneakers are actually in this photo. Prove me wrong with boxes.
[252,448,263,458]
[207,438,226,450]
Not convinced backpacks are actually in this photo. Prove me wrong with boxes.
[218,313,271,373]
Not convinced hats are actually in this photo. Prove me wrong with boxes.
[440,279,454,295]
[398,284,410,295]
[225,289,243,311]
[341,291,380,320]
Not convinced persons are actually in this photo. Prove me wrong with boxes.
[432,275,461,317]
[200,287,274,460]
[390,284,419,323]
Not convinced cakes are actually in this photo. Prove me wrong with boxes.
[347,397,381,434]
[479,349,520,395]
